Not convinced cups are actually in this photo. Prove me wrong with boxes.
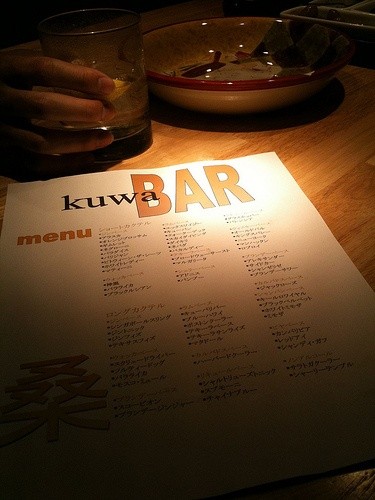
[37,10,153,165]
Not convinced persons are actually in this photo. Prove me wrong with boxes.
[0,48,118,172]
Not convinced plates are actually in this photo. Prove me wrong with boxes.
[141,17,355,115]
[280,0,375,45]
